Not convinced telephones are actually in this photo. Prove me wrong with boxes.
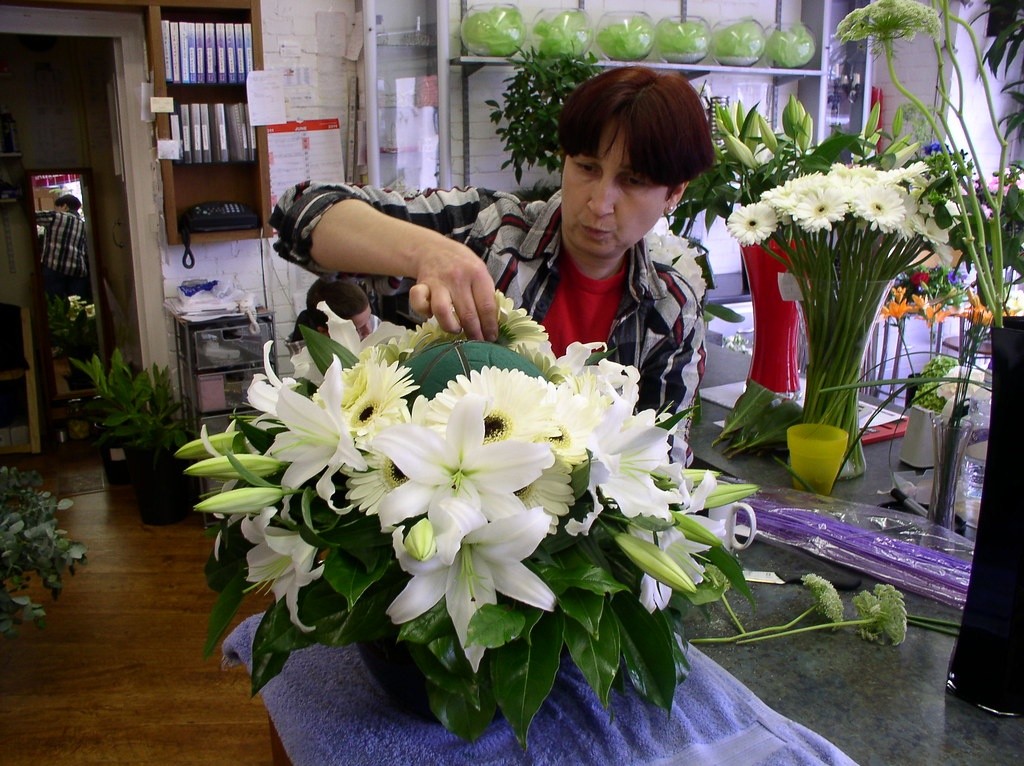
[238,290,253,312]
[183,200,259,232]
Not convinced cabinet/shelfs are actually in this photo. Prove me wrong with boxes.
[0,1,277,501]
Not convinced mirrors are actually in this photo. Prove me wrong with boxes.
[28,168,111,401]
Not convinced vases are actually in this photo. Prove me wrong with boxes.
[348,585,537,734]
[726,227,806,399]
[896,403,946,470]
[914,416,972,535]
[766,272,888,479]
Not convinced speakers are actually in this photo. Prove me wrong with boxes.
[987,5,1024,38]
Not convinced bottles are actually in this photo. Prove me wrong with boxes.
[0,105,21,153]
[956,369,991,503]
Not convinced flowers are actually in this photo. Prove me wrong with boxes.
[191,0,1024,748]
[43,293,100,349]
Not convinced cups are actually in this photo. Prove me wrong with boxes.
[690,480,756,555]
[786,423,849,495]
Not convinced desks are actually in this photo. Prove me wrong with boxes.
[671,328,1024,766]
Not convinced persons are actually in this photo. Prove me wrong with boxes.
[33,196,86,356]
[269,69,716,467]
[283,276,383,359]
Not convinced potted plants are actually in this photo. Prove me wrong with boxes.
[72,349,137,491]
[117,367,202,525]
